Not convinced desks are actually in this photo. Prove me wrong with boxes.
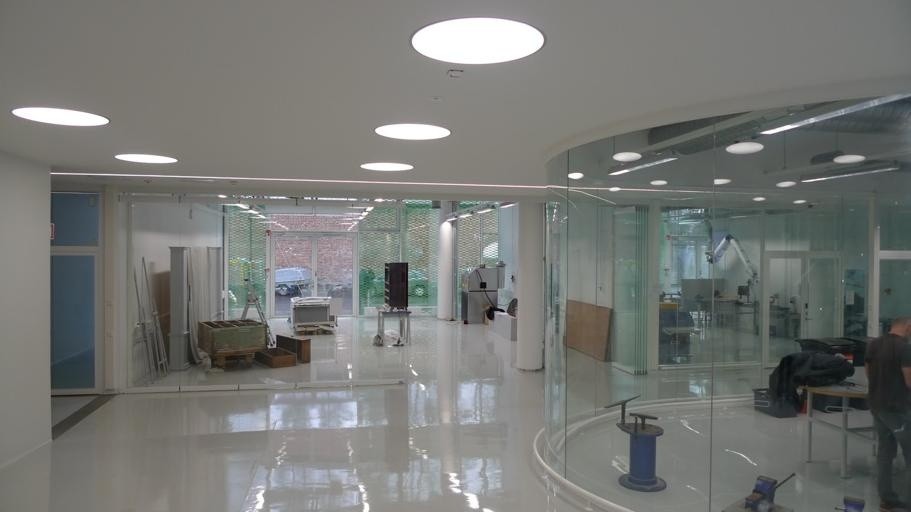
[799,386,876,479]
[378,310,411,344]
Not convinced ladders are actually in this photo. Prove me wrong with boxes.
[241,293,275,347]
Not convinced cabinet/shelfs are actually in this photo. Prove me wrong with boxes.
[697,298,800,339]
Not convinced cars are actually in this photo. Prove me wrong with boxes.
[326,268,429,298]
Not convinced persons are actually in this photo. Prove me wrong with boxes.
[864,315,911,512]
